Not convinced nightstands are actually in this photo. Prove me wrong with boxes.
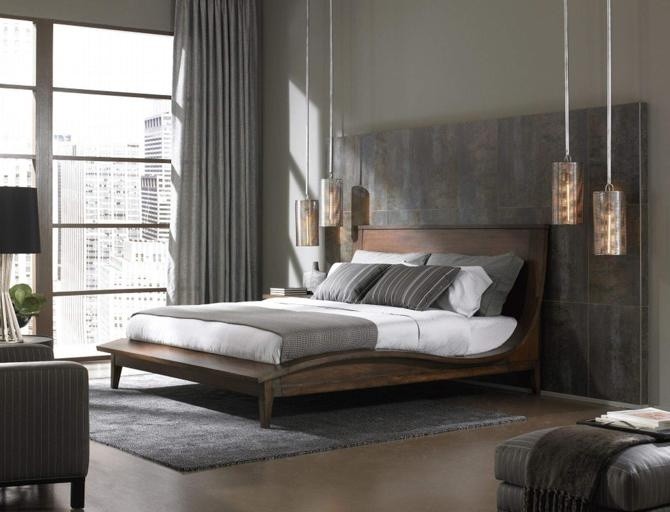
[261,293,313,301]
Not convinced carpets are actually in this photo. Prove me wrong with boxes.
[88,361,525,473]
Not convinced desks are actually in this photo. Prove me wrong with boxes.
[22,336,53,345]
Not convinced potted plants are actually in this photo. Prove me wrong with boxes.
[6,283,47,328]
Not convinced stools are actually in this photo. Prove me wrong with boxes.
[493,420,670,511]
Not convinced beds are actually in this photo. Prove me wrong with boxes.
[96,223,550,429]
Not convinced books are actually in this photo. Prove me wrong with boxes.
[269,287,309,295]
[595,405,670,432]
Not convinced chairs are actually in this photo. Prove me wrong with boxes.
[0,342,89,512]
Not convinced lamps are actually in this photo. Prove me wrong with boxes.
[0,186,42,344]
[552,1,585,225]
[319,1,343,227]
[592,2,627,256]
[295,0,320,247]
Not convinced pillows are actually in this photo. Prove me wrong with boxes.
[310,262,389,303]
[425,253,524,316]
[352,250,431,265]
[360,263,461,311]
[403,261,494,318]
